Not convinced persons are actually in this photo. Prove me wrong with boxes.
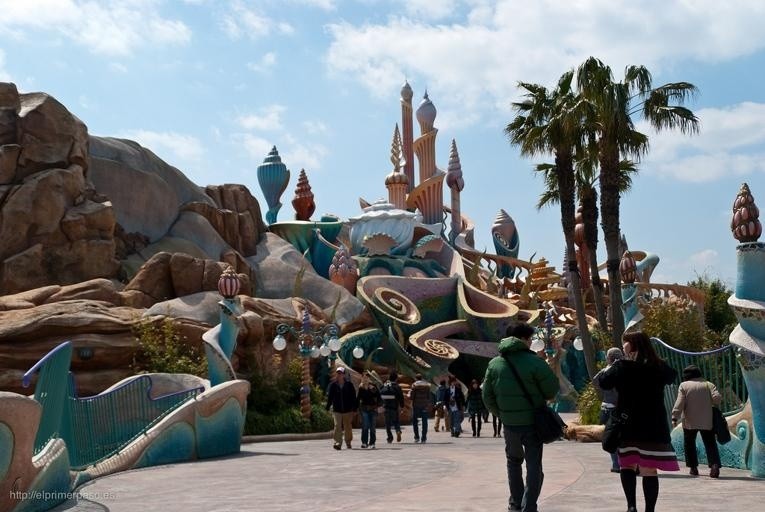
[598,330,680,512]
[324,366,502,450]
[592,346,623,472]
[671,364,723,479]
[481,322,561,512]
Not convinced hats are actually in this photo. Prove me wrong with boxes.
[336,367,345,373]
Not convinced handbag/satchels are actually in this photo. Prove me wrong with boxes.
[381,386,396,399]
[603,408,628,454]
[712,405,731,445]
[533,407,562,444]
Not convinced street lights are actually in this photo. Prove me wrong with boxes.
[531,306,587,411]
[272,303,342,429]
[326,327,364,385]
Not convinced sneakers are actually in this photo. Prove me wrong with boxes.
[710,464,719,478]
[334,445,341,450]
[347,442,351,448]
[397,431,401,442]
[388,437,392,442]
[690,467,698,475]
[361,443,368,448]
[369,445,375,448]
[508,503,522,512]
